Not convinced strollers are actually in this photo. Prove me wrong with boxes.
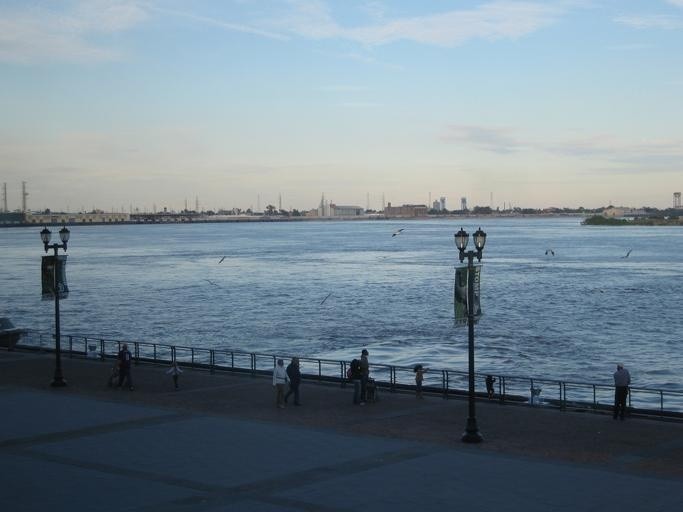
[366,377,380,404]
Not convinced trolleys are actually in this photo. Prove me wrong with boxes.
[108,362,124,389]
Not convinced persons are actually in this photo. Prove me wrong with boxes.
[348,358,363,405]
[359,347,369,402]
[285,356,301,406]
[612,362,630,422]
[164,362,182,390]
[113,344,134,391]
[485,375,496,398]
[412,364,428,397]
[272,358,290,409]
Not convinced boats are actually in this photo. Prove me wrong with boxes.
[0,317,23,347]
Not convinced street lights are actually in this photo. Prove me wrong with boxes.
[37,222,72,391]
[452,225,492,443]
